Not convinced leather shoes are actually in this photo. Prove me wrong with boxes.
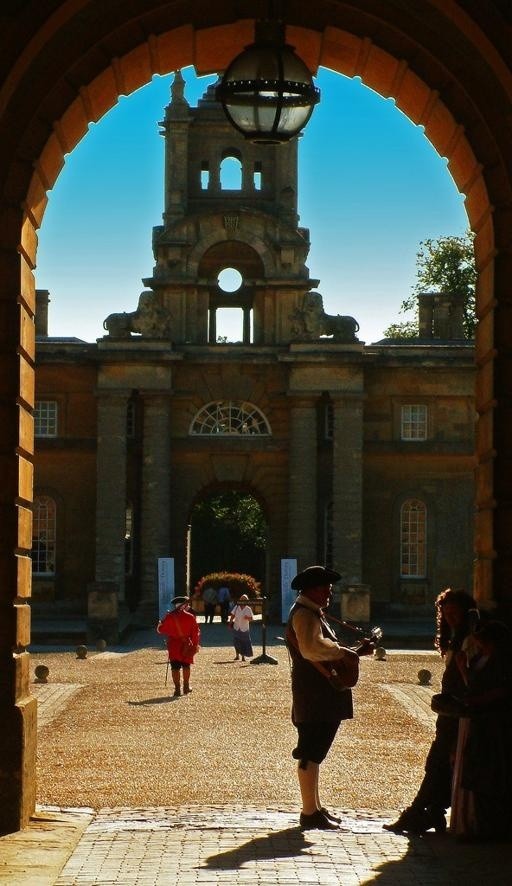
[300,808,341,829]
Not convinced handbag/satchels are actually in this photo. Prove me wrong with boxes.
[227,623,233,630]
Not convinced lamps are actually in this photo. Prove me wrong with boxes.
[215,19,320,147]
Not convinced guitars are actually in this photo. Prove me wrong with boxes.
[326,626,383,692]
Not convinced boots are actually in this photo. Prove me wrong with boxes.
[172,672,180,696]
[183,668,192,694]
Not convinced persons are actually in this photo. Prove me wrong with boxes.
[217,586,231,624]
[157,597,200,696]
[285,566,376,829]
[382,588,477,833]
[445,606,512,839]
[203,587,217,624]
[229,594,253,661]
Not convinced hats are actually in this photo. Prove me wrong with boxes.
[292,566,341,590]
[171,597,188,605]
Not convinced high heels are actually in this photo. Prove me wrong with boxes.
[382,805,447,834]
